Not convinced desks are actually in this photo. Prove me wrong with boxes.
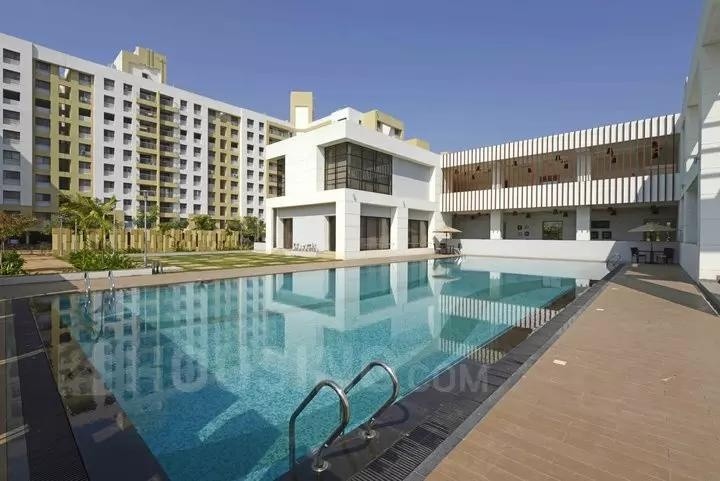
[442,244,454,255]
[636,249,666,264]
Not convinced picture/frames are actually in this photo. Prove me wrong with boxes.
[517,224,530,236]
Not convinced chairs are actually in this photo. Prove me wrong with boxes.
[434,243,442,254]
[655,247,672,264]
[453,243,462,254]
[661,248,674,263]
[631,246,646,264]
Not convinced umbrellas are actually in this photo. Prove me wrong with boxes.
[627,221,677,251]
[433,225,463,254]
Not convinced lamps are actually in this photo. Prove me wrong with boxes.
[454,140,662,180]
